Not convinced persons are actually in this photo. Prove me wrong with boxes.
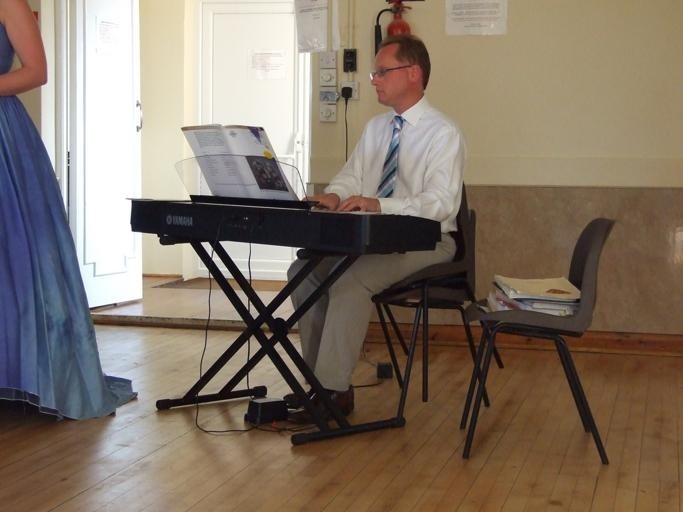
[282,33,466,424]
[1,0,138,422]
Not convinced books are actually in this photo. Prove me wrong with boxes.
[181,123,300,201]
[477,274,582,318]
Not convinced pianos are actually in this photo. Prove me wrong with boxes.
[131,200,441,253]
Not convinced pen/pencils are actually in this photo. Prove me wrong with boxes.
[496,297,522,311]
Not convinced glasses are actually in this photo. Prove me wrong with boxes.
[368,63,415,81]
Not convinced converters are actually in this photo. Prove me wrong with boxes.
[245,396,288,424]
[377,361,394,378]
[341,87,352,99]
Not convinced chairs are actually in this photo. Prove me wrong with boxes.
[458,217,618,465]
[371,181,504,422]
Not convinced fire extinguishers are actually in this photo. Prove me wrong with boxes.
[375,0,411,54]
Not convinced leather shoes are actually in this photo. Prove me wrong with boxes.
[283,382,317,401]
[289,386,356,423]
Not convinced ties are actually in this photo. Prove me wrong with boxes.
[375,115,405,198]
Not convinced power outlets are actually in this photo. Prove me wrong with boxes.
[340,80,360,100]
